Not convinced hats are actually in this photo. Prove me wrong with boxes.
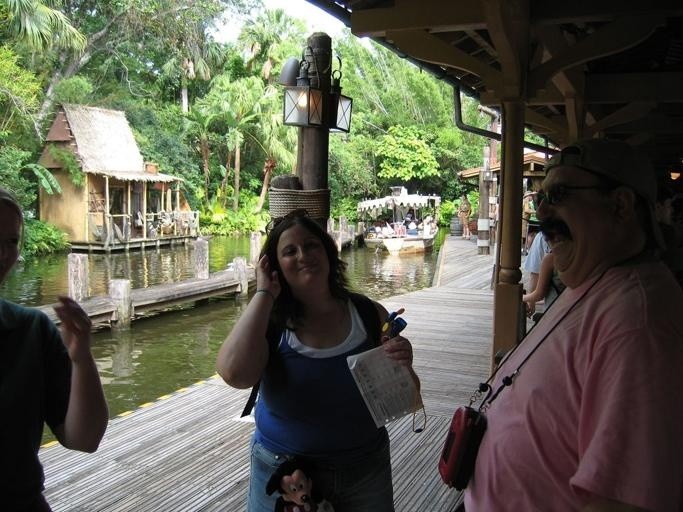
[542,136,667,253]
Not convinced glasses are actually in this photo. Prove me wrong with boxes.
[532,182,606,207]
[265,208,309,238]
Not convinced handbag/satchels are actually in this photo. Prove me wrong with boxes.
[437,404,489,493]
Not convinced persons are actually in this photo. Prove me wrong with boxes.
[520,189,538,257]
[1,185,111,512]
[462,120,683,511]
[404,213,415,228]
[518,246,564,320]
[518,227,552,296]
[213,203,424,510]
[417,215,436,232]
[457,193,471,240]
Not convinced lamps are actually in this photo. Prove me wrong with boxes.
[278,45,353,135]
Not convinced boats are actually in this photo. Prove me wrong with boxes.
[364,225,440,256]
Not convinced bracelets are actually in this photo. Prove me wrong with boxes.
[255,288,277,303]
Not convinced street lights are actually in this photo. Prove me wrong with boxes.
[280,31,353,236]
[477,169,498,254]
